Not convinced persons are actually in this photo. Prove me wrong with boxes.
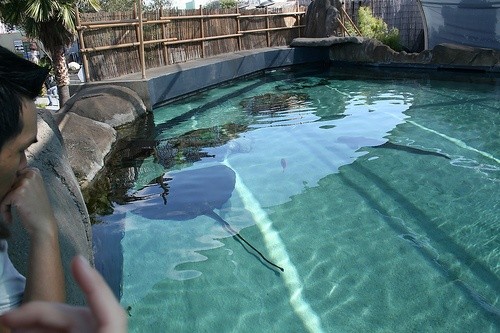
[0,45,126,333]
[44,74,59,106]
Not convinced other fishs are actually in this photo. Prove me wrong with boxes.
[122,68,316,278]
